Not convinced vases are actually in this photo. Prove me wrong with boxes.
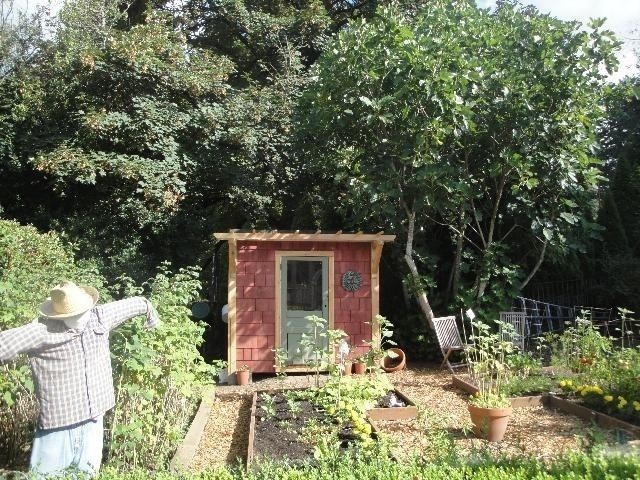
[354,363,365,375]
[379,348,407,372]
[343,363,352,376]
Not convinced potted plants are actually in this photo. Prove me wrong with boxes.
[460,319,519,443]
[236,364,249,385]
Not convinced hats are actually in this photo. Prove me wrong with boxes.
[39,280,99,319]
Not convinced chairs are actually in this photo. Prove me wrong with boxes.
[498,311,525,352]
[431,316,466,374]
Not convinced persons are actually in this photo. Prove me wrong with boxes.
[0,281,162,479]
